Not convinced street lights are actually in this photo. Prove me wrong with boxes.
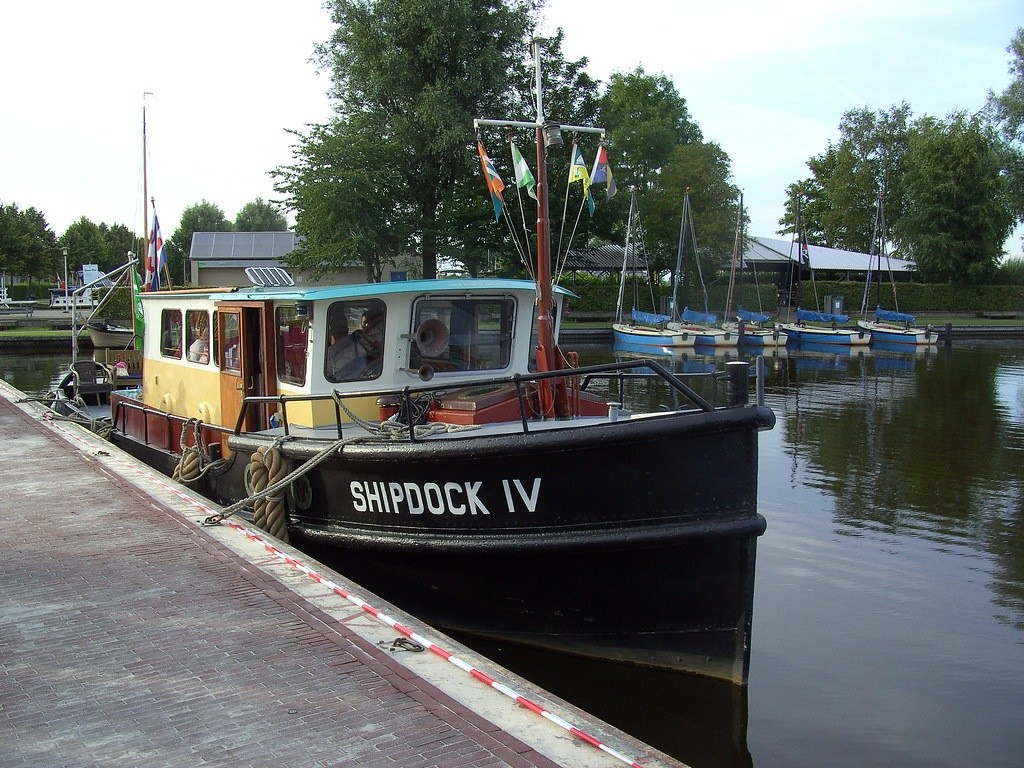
[59,247,71,313]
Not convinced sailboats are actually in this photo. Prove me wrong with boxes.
[856,193,939,345]
[720,188,788,345]
[774,191,872,344]
[665,188,741,345]
[611,185,697,346]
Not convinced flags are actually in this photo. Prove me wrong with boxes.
[512,144,536,200]
[568,144,595,217]
[591,148,617,203]
[132,266,145,350]
[145,208,167,292]
[478,142,504,223]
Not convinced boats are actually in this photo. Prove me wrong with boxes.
[50,28,778,688]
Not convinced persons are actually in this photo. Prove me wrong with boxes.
[352,310,381,355]
[327,317,366,379]
[172,327,208,362]
